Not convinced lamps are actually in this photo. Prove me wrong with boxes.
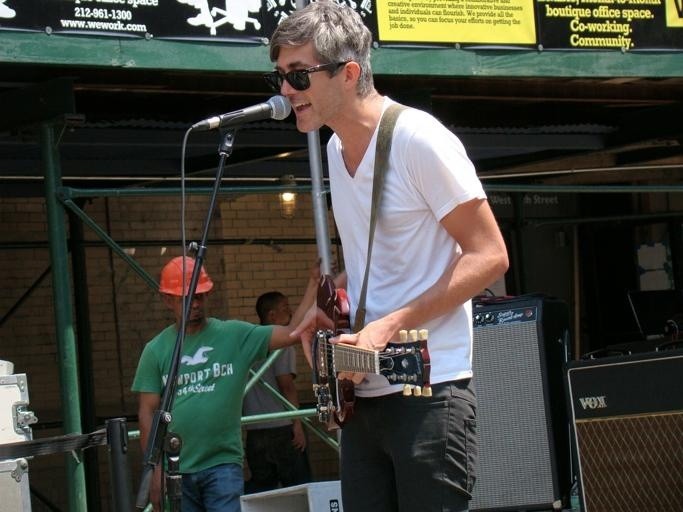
[277,173,298,220]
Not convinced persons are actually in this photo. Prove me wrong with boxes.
[240,290,316,492]
[129,255,338,511]
[266,0,515,512]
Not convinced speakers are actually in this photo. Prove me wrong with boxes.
[467,301,562,512]
[563,348,683,512]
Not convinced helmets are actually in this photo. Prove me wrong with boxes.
[159,256,215,298]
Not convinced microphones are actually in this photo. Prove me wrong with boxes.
[208,95,290,129]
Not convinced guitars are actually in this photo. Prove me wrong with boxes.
[308,274,433,434]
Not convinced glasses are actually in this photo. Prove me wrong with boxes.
[262,62,342,91]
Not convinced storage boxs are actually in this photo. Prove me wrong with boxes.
[239,480,344,512]
